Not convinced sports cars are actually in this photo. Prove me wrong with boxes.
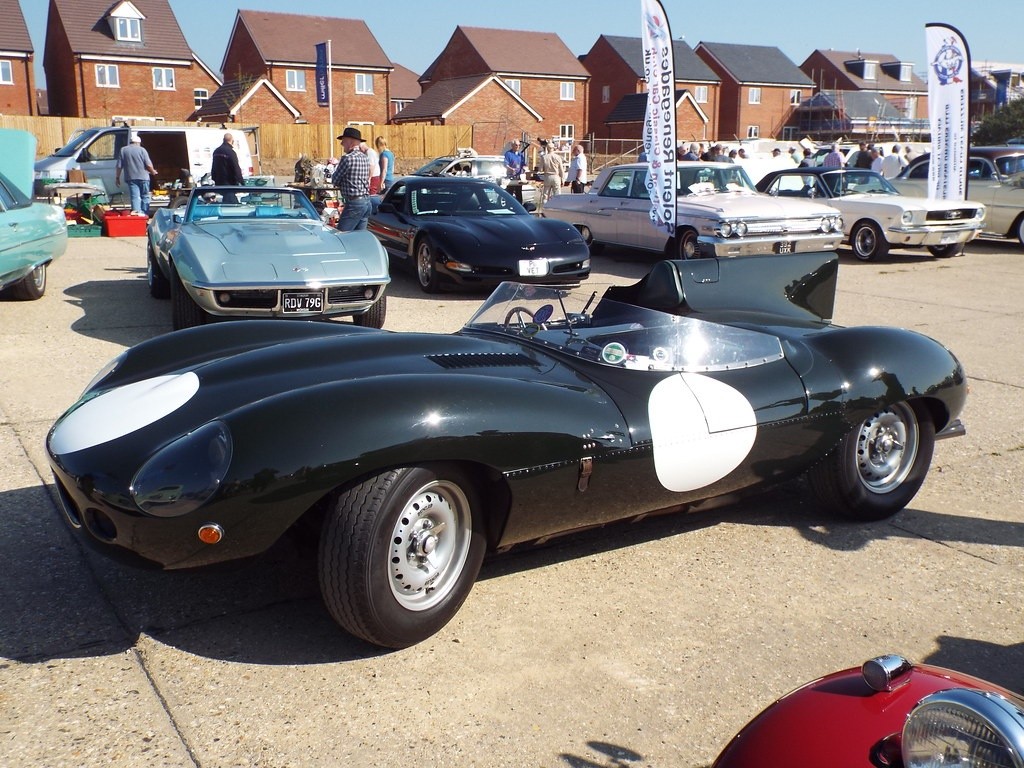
[45,251,969,648]
[146,185,389,332]
[370,175,592,295]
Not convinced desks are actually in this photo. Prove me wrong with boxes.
[506,180,594,213]
[48,188,95,220]
[290,185,340,209]
[170,188,192,201]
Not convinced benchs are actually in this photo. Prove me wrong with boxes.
[778,190,809,197]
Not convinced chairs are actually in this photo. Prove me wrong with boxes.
[463,165,470,176]
[458,191,480,210]
[194,205,222,219]
[256,206,284,216]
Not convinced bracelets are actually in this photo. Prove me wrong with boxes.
[116,177,120,179]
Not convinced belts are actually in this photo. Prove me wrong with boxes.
[350,196,364,200]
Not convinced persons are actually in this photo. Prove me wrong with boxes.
[115,135,158,214]
[567,144,587,193]
[246,191,264,205]
[332,127,373,231]
[539,142,565,205]
[771,144,802,171]
[359,140,380,195]
[503,139,526,206]
[676,140,749,191]
[797,148,817,188]
[211,133,245,204]
[638,150,648,162]
[375,136,395,195]
[853,141,929,181]
[823,143,846,192]
[200,184,217,204]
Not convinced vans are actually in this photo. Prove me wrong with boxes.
[34,126,262,211]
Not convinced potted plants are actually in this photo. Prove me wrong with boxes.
[35,178,58,197]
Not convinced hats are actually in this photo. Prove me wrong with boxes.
[337,128,367,142]
[771,148,781,152]
[130,135,141,142]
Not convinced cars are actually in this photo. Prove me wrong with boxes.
[811,144,900,168]
[0,128,67,301]
[707,654,1024,768]
[753,167,988,260]
[399,153,536,207]
[541,160,846,260]
[855,143,1023,248]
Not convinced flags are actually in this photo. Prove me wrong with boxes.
[642,0,676,240]
[925,23,971,202]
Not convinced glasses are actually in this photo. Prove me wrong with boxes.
[513,144,521,146]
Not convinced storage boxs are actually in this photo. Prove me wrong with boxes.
[103,216,148,236]
[67,170,84,182]
[68,224,101,237]
